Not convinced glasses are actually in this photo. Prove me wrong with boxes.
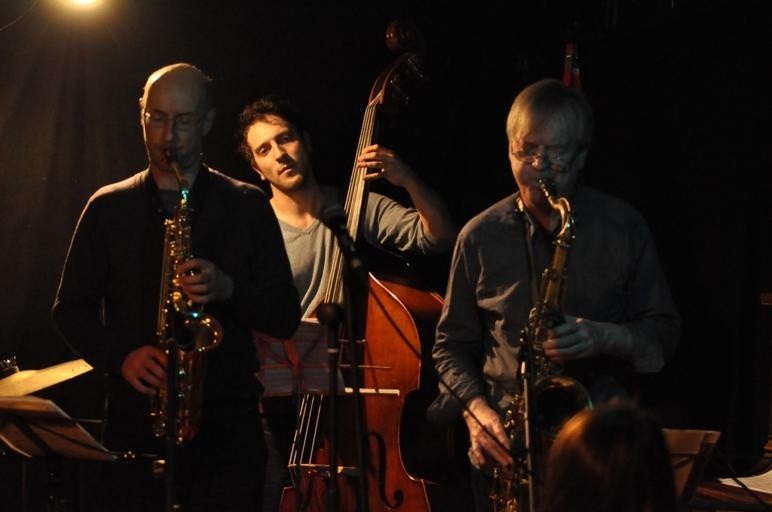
[509,142,573,164]
[140,111,205,130]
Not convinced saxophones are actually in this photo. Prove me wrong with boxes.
[488,175,595,511]
[149,149,224,456]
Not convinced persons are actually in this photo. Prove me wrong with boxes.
[52,63,301,512]
[430,78,677,512]
[236,94,453,512]
[534,395,676,512]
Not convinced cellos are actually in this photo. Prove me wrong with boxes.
[280,16,445,510]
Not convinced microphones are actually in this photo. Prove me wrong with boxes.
[165,302,196,352]
[317,200,371,292]
[517,330,537,382]
